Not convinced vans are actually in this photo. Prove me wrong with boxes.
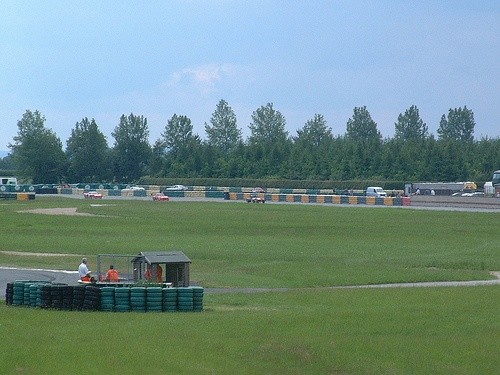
[366,186,386,196]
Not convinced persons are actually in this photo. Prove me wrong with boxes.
[374,189,378,197]
[344,188,348,194]
[350,189,353,196]
[145,263,162,282]
[495,191,500,198]
[79,258,93,281]
[424,189,430,196]
[85,277,98,287]
[106,265,119,283]
[415,187,420,196]
[431,188,435,196]
[385,191,400,197]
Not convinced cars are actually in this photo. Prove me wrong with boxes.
[82,190,103,199]
[152,192,171,200]
[164,184,189,191]
[246,194,265,202]
